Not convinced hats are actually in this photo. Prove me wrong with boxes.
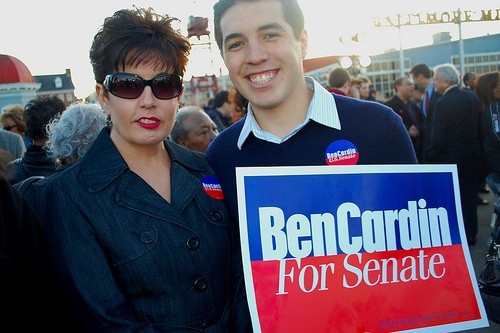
[212,91,228,108]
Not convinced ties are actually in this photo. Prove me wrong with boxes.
[425,91,430,120]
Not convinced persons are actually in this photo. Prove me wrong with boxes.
[198,1,417,333]
[0,62,500,333]
[25,4,253,333]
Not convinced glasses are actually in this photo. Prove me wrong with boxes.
[103,73,183,100]
[3,125,17,132]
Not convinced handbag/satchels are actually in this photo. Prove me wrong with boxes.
[478,254,500,324]
